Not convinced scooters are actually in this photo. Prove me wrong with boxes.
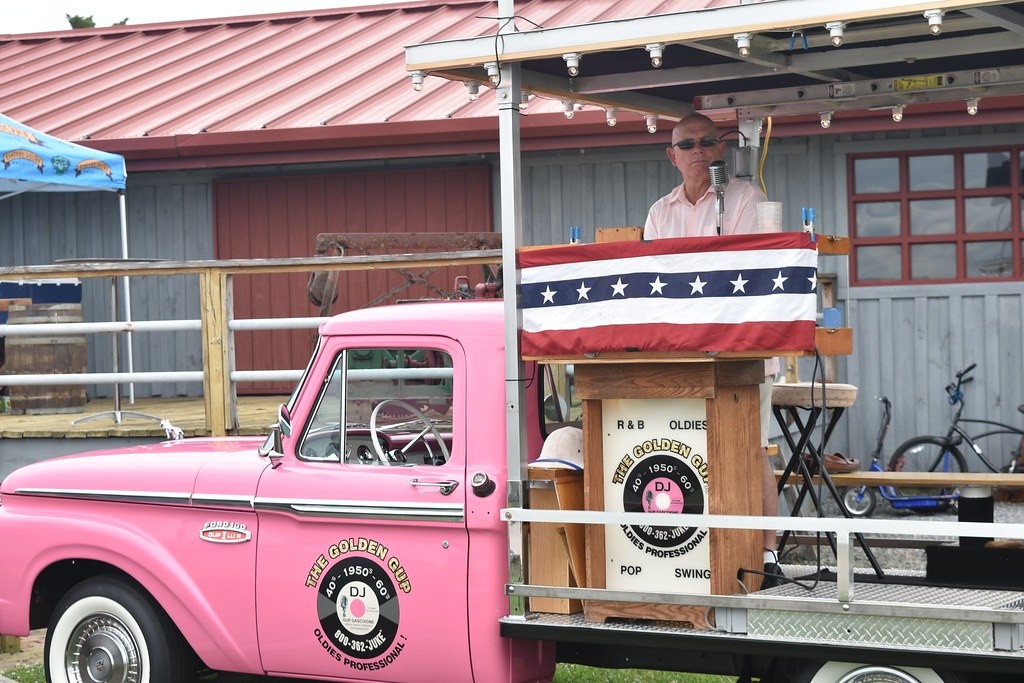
[842,397,964,516]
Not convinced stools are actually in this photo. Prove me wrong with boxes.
[770,379,886,578]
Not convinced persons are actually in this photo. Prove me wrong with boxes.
[643,115,785,590]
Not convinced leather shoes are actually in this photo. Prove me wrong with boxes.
[815,453,862,477]
[792,452,814,475]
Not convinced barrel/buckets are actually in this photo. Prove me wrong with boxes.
[957,482,993,548]
[6,302,93,413]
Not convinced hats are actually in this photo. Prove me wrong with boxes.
[528,426,584,471]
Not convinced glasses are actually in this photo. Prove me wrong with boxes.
[671,137,720,150]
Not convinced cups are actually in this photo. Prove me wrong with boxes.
[756,201,784,234]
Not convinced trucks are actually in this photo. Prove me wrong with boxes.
[0,296,1024,683]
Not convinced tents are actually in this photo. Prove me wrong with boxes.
[0,115,135,403]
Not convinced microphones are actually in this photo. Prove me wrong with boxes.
[709,160,729,235]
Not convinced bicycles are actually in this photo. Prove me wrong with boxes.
[888,363,1024,513]
